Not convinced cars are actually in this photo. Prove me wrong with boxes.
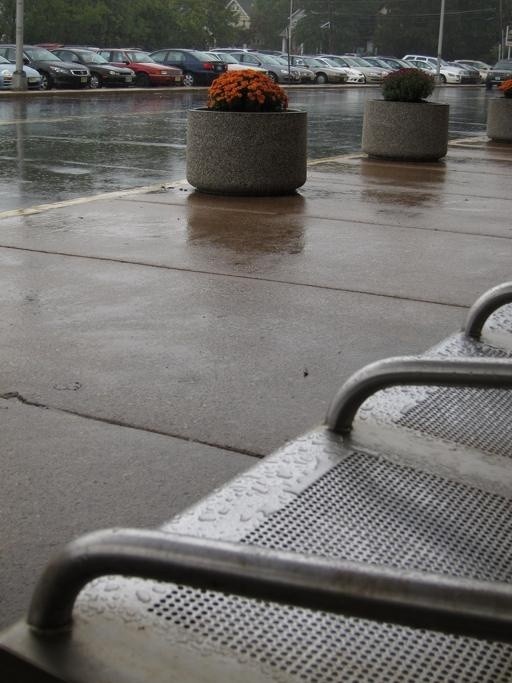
[0,54,42,91]
[0,41,93,92]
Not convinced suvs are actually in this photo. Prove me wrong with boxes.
[484,58,512,89]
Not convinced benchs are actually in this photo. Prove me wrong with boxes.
[2,274,511,682]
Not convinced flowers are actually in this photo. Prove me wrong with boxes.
[498,77,512,97]
[378,67,437,99]
[198,67,292,110]
